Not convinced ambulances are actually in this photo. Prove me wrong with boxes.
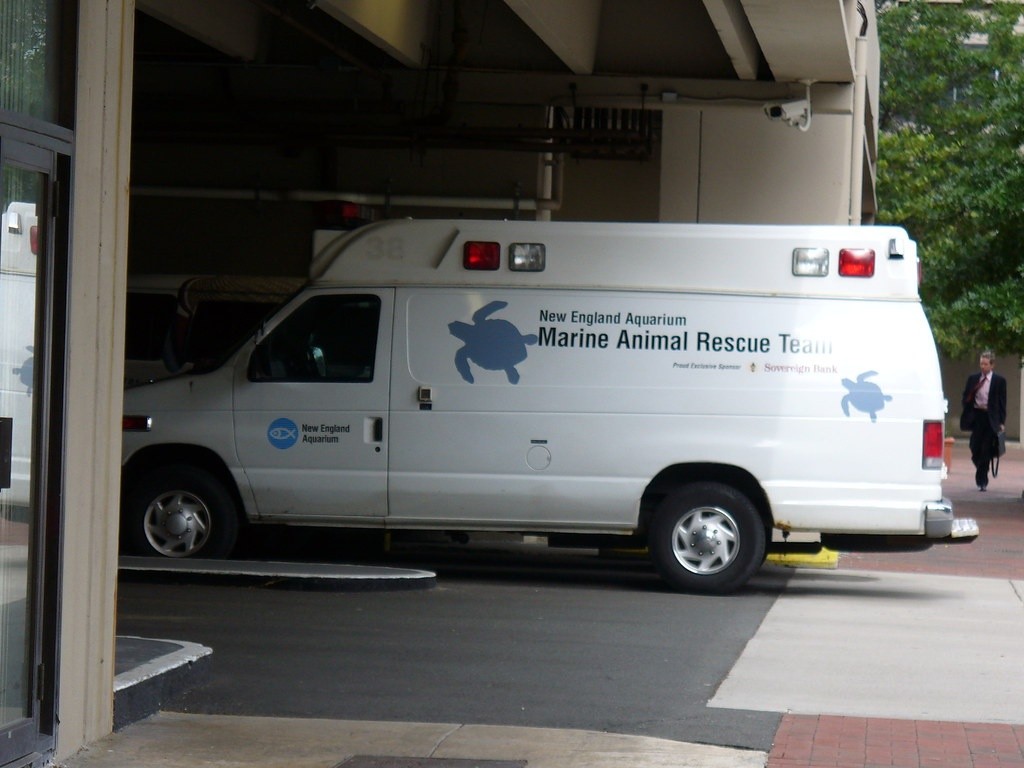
[121,216,979,592]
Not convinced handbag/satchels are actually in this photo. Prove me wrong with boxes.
[991,428,1006,478]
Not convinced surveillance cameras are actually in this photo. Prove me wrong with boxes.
[761,99,808,121]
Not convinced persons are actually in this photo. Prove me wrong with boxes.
[960,352,1006,491]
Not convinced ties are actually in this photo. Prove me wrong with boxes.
[964,376,986,405]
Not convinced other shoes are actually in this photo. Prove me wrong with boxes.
[980,485,986,492]
